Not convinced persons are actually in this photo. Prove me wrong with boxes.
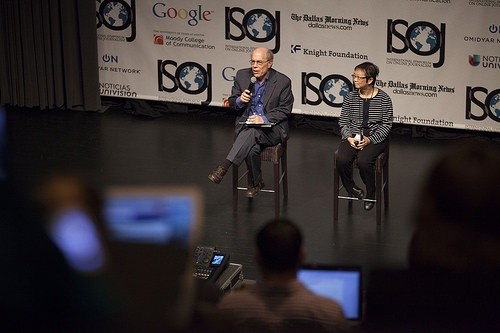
[367,139,500,333]
[337,63,393,211]
[208,46,294,198]
[214,218,350,333]
[0,111,117,333]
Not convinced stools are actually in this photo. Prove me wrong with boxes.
[333,137,389,225]
[233,144,288,216]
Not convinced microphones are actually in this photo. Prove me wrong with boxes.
[246,76,256,94]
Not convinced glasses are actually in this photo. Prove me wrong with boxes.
[351,75,366,80]
[249,60,270,65]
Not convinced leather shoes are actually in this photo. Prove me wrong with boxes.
[207,161,228,184]
[246,178,265,197]
[364,187,376,211]
[350,185,364,198]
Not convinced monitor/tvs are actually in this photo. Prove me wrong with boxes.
[297,264,362,320]
[99,184,206,333]
